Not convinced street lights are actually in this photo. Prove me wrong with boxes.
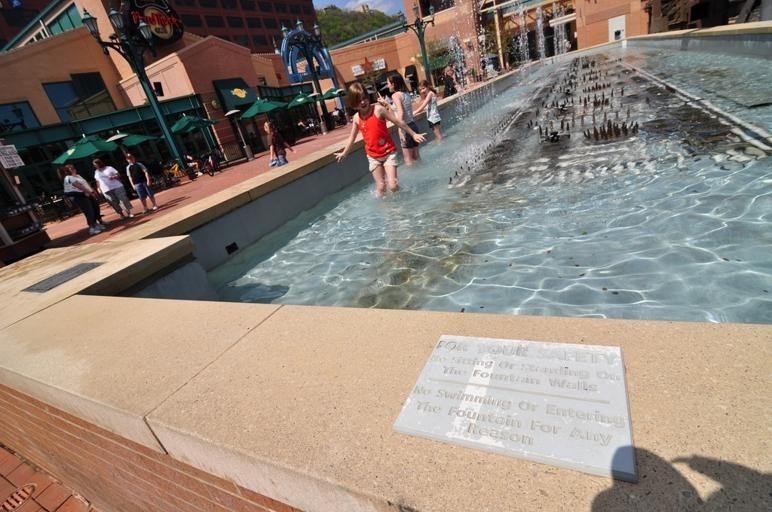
[281,19,333,131]
[82,7,189,171]
[400,4,434,86]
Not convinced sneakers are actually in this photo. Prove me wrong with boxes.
[89,224,106,235]
[120,214,135,220]
[143,206,158,214]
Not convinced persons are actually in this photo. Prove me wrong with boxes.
[334,69,443,196]
[296,117,310,135]
[442,63,460,98]
[56,153,158,234]
[263,120,294,167]
[181,148,203,177]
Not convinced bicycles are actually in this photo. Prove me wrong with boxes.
[201,150,220,176]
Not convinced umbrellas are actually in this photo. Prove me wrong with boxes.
[238,96,289,121]
[50,130,159,165]
[160,112,220,153]
[285,88,349,111]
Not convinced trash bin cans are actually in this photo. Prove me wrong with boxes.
[467,70,474,84]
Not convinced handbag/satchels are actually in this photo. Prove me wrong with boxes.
[268,160,277,167]
